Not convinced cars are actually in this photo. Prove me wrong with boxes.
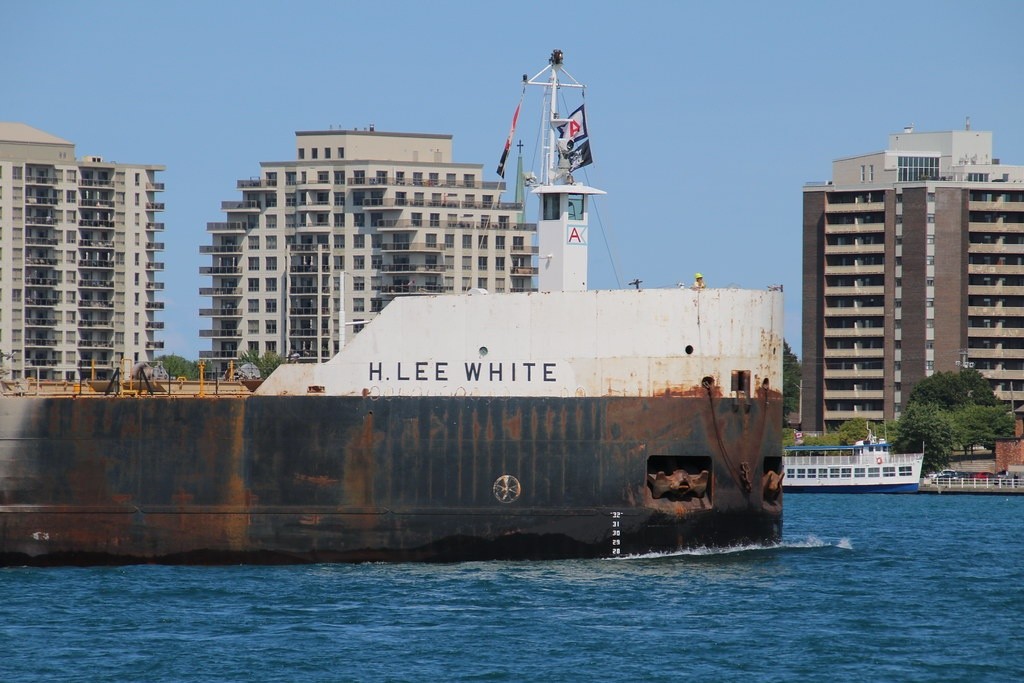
[925,468,1010,486]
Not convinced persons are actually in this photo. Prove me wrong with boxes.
[692,272,706,289]
[1013,472,1020,488]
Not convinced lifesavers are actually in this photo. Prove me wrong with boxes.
[876,456,882,464]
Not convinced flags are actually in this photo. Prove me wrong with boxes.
[495,100,519,177]
[557,104,593,172]
[795,432,803,438]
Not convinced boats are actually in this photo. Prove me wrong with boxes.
[783,419,925,494]
[0,49,783,567]
